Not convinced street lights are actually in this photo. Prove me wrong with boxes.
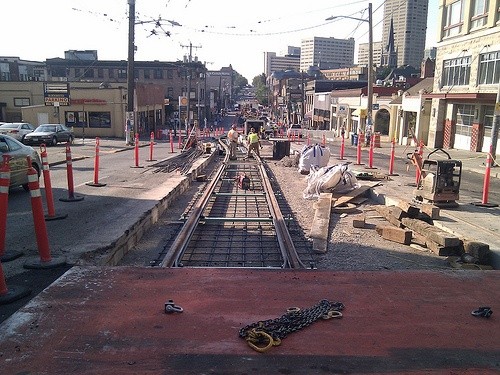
[124,0,182,145]
[325,2,373,145]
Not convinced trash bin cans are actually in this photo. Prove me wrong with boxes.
[351,133,358,146]
[155,130,163,140]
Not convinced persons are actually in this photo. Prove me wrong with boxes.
[227,124,239,159]
[247,127,261,158]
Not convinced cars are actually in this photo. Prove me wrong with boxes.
[287,124,303,138]
[0,123,35,143]
[217,83,283,134]
[24,123,75,146]
[0,134,41,191]
